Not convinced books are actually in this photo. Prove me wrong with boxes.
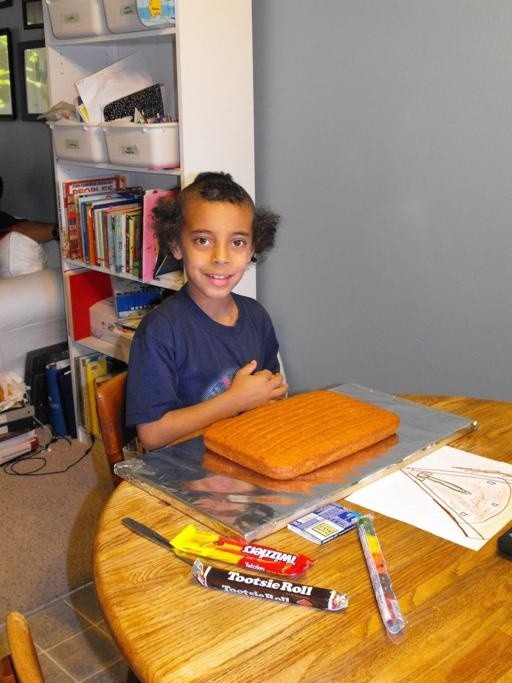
[73,351,130,437]
[0,341,79,465]
[64,267,161,340]
[64,176,182,283]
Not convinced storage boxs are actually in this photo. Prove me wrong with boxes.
[44,115,179,169]
[47,0,175,38]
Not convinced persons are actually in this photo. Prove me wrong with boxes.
[0,211,60,243]
[120,168,292,451]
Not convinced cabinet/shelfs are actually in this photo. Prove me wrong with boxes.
[40,0,256,448]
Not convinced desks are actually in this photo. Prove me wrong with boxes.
[94,390,511,681]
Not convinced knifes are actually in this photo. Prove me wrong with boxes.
[121,517,221,571]
